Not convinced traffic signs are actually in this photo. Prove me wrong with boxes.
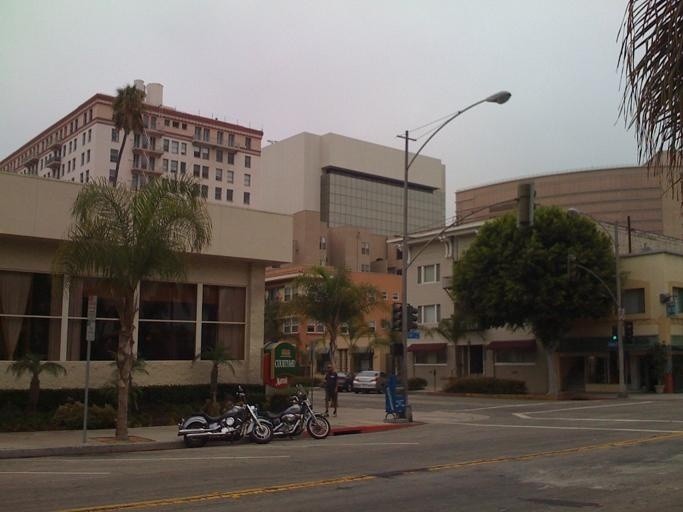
[406,331,421,340]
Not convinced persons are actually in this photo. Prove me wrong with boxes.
[319,361,340,420]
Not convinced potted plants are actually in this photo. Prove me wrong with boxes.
[644,338,673,395]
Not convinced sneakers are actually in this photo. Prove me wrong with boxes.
[323,412,329,417]
[332,412,337,416]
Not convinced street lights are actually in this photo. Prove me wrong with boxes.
[395,90,520,423]
[565,206,630,399]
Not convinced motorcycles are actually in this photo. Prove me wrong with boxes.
[258,384,334,439]
[174,384,275,444]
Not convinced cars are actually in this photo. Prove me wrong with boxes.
[352,371,391,395]
[332,370,357,393]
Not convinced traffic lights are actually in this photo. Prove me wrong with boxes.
[611,323,618,341]
[392,302,405,332]
[406,302,419,330]
[624,320,634,342]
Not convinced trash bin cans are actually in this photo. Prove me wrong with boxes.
[383,373,406,424]
